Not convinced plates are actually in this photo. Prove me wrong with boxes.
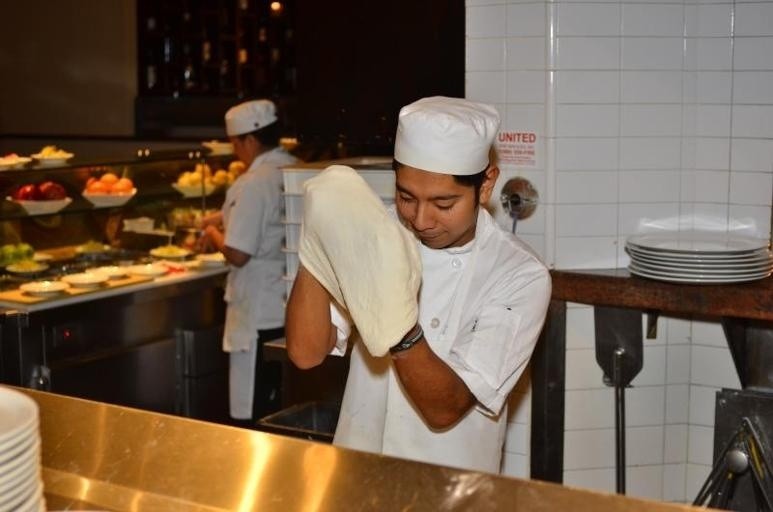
[202,141,233,154]
[0,154,74,170]
[149,248,190,261]
[0,388,47,512]
[624,230,773,284]
[128,265,169,277]
[80,187,137,208]
[171,184,217,198]
[5,243,111,275]
[5,196,72,214]
[20,265,128,299]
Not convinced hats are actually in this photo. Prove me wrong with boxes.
[393,94,500,177]
[225,98,278,137]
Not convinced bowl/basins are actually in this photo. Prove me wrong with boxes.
[176,226,223,253]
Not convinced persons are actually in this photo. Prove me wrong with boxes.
[284,97,553,474]
[198,98,304,421]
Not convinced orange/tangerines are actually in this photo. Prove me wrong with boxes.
[0,243,33,267]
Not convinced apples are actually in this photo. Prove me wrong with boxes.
[13,181,65,201]
[178,160,247,188]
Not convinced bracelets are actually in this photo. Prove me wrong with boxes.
[383,326,425,354]
